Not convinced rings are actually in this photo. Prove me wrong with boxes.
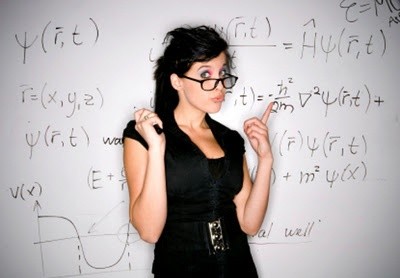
[144,115,149,119]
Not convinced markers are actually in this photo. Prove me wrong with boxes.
[133,106,162,135]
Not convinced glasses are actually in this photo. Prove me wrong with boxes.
[182,72,238,91]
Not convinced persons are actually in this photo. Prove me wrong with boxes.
[122,25,275,278]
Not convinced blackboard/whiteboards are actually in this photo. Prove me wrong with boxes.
[1,0,399,278]
